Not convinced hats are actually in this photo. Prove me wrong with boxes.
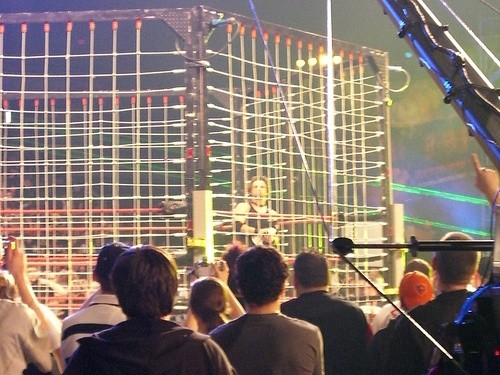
[399,271,433,309]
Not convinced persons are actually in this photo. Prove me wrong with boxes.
[206,245,324,375]
[227,175,282,250]
[279,249,373,375]
[187,261,245,336]
[221,245,249,309]
[0,268,23,300]
[0,236,62,375]
[359,153,500,375]
[61,245,236,375]
[369,257,431,336]
[398,270,435,311]
[62,241,131,375]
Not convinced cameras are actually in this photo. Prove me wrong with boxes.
[194,262,222,278]
[1,239,15,257]
